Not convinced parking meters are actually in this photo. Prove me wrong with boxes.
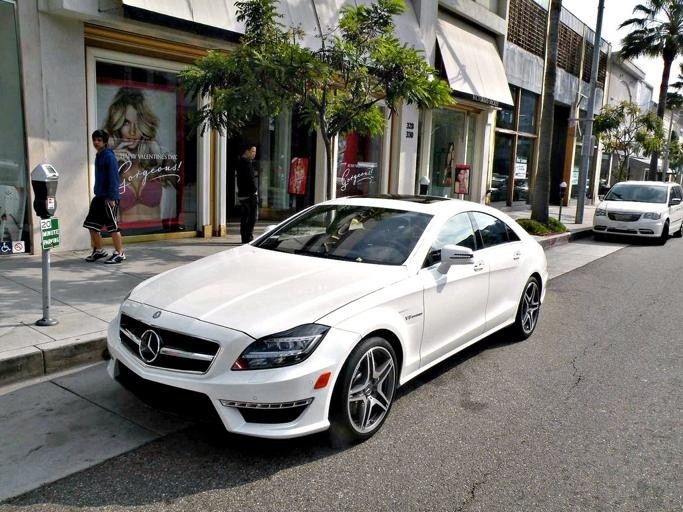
[557,181,570,223]
[416,173,432,196]
[29,159,67,328]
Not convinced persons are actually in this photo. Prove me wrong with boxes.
[101,86,177,223]
[83,130,126,264]
[236,145,258,243]
[455,168,468,193]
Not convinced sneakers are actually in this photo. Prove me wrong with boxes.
[104,250,126,265]
[84,247,108,262]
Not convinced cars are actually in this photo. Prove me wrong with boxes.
[247,158,377,198]
[100,187,553,460]
[573,176,607,200]
[589,178,683,249]
[0,158,30,251]
[489,173,528,203]
